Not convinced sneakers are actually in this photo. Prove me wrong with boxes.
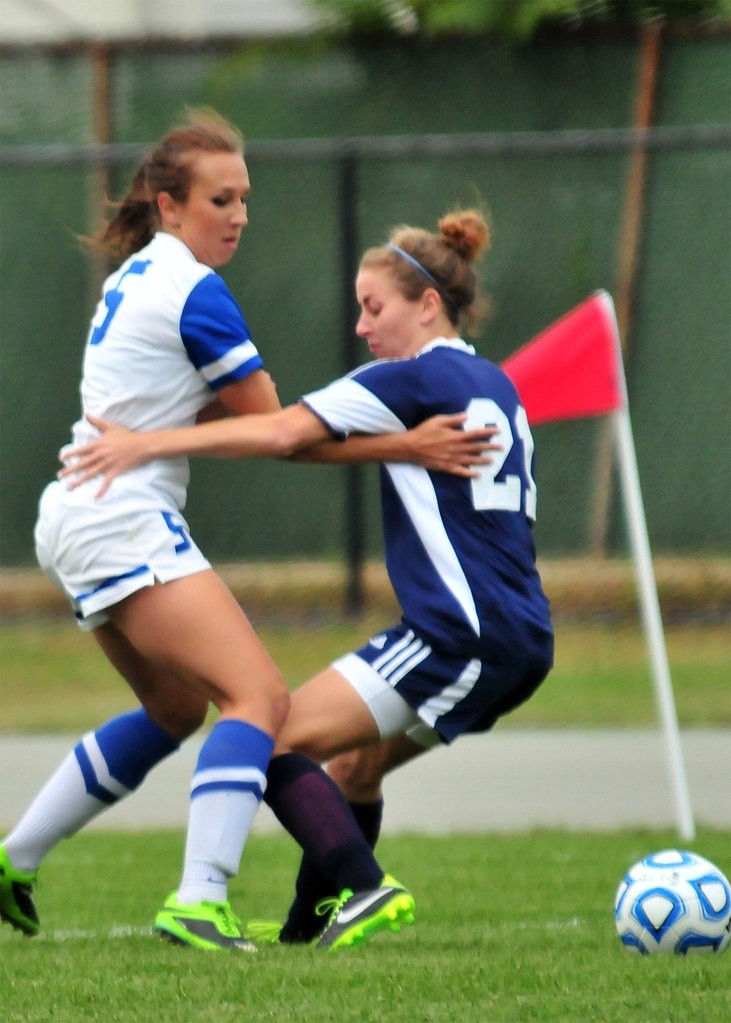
[155,892,257,954]
[244,921,319,946]
[0,845,40,936]
[315,873,415,958]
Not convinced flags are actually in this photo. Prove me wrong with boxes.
[499,294,615,428]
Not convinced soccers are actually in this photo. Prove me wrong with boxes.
[610,847,731,960]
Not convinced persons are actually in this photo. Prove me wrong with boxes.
[56,205,555,950]
[1,116,503,952]
